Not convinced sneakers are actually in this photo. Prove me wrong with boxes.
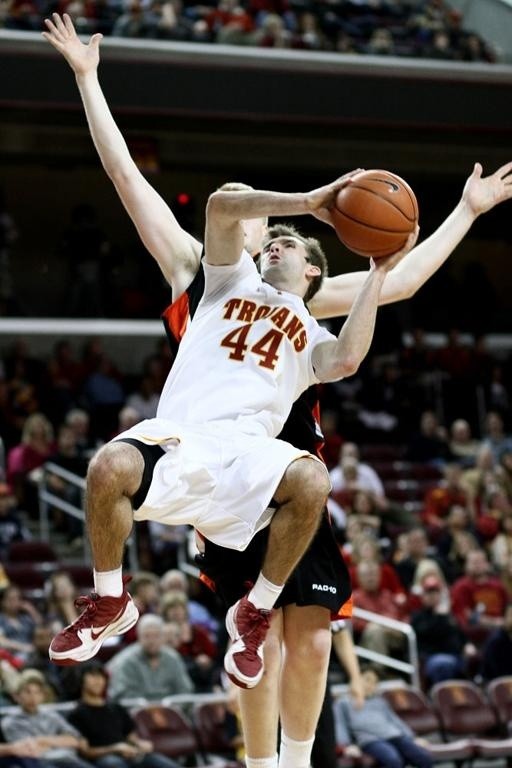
[224,581,272,689]
[48,574,140,667]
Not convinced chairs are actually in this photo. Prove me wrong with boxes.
[340,678,511,767]
[133,701,246,768]
[370,443,435,511]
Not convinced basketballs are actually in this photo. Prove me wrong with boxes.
[333,169,421,257]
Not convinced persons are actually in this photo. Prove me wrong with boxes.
[46,165,422,691]
[0,335,511,767]
[0,0,512,63]
[41,12,512,767]
